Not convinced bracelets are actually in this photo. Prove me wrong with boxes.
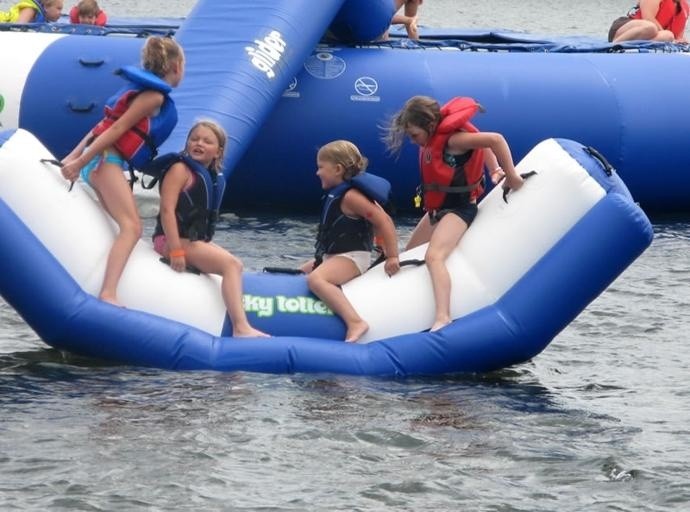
[488,166,502,176]
[170,249,186,256]
[375,237,385,244]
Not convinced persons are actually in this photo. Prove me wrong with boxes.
[608,0,690,46]
[375,97,526,333]
[60,35,186,308]
[70,0,107,27]
[389,1,424,39]
[153,118,272,338]
[0,0,63,25]
[295,139,401,345]
